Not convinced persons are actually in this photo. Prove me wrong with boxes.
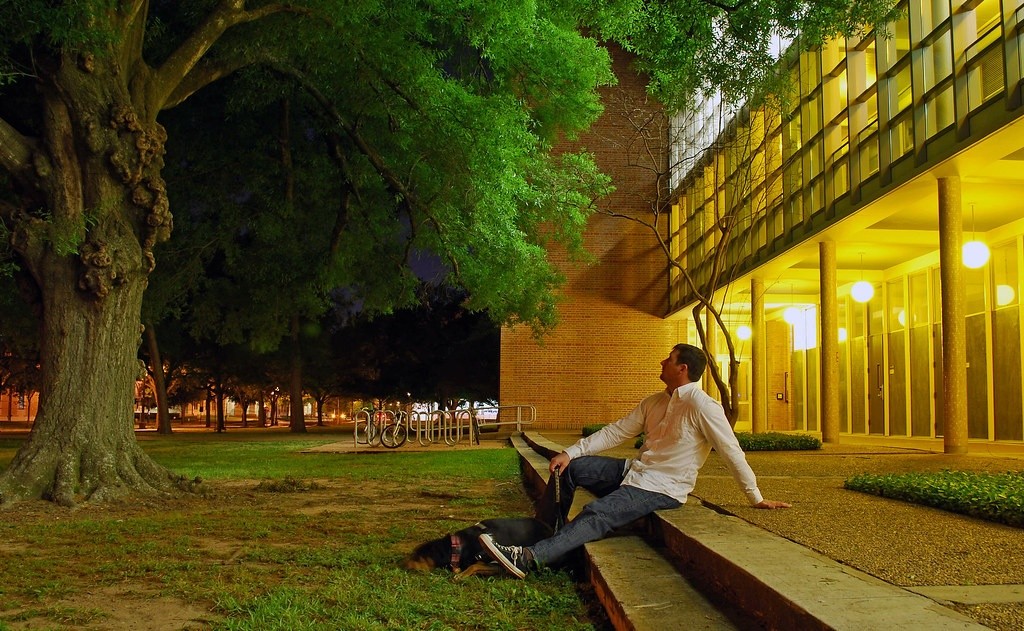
[476,340,795,581]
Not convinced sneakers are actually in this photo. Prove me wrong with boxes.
[479,534,529,580]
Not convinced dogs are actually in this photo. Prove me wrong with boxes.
[396,517,555,582]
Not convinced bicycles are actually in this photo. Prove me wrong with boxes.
[379,412,407,448]
[353,407,381,444]
[470,405,482,444]
